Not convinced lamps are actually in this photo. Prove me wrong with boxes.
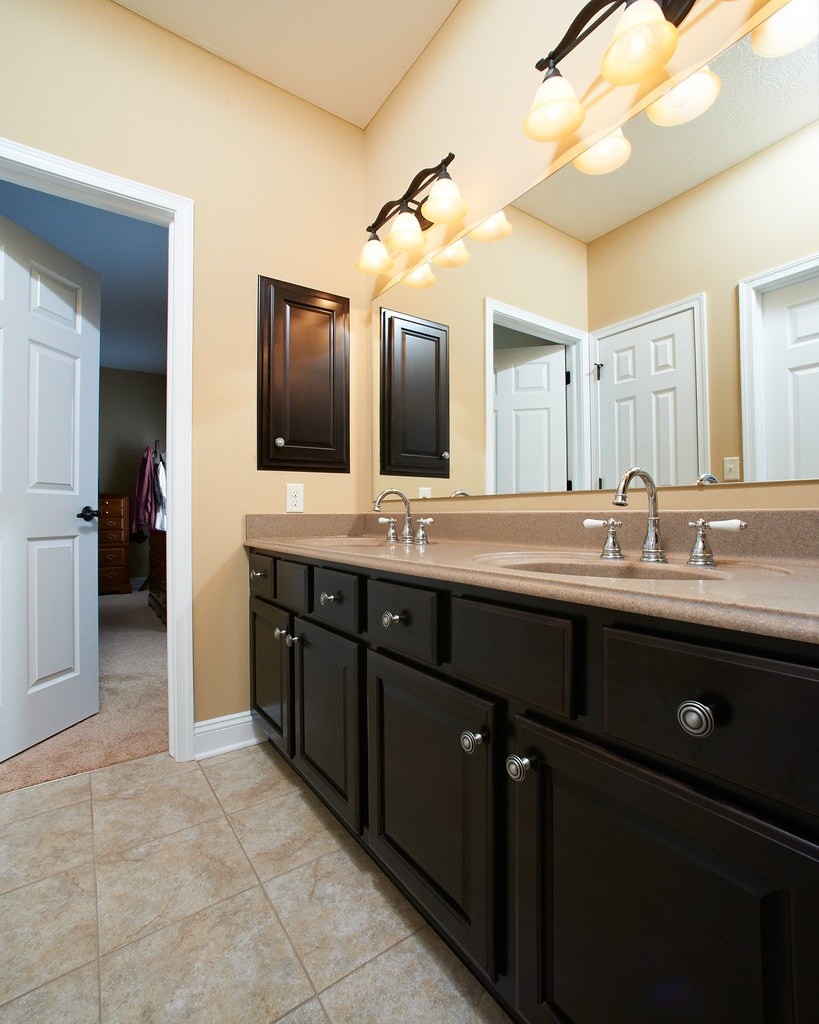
[573,0,819,174]
[355,151,470,274]
[402,209,513,288]
[524,0,696,146]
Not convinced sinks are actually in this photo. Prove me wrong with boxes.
[472,550,792,582]
[295,538,391,547]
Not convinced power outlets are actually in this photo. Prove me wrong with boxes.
[418,488,431,498]
[286,483,304,513]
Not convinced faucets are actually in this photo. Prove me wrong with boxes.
[373,488,414,542]
[450,490,470,497]
[612,466,667,562]
[695,473,719,485]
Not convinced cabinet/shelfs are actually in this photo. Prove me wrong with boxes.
[241,511,819,1024]
[258,274,350,474]
[99,495,132,594]
[146,529,167,626]
[380,307,450,480]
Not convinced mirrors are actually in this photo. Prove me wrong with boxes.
[375,0,819,503]
